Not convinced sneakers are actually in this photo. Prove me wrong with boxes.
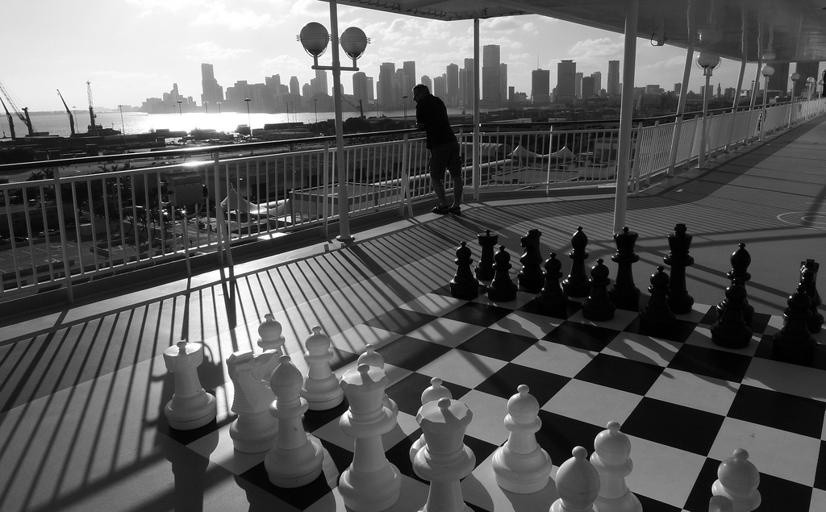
[448,207,461,215]
[431,205,448,214]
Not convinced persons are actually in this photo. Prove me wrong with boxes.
[412,84,464,214]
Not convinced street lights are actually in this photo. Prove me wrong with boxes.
[402,96,409,128]
[244,97,254,156]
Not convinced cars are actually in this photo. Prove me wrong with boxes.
[6,236,28,242]
[38,228,60,237]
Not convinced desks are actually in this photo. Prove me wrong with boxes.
[154,271,826,512]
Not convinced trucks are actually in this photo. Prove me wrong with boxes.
[0,255,74,289]
